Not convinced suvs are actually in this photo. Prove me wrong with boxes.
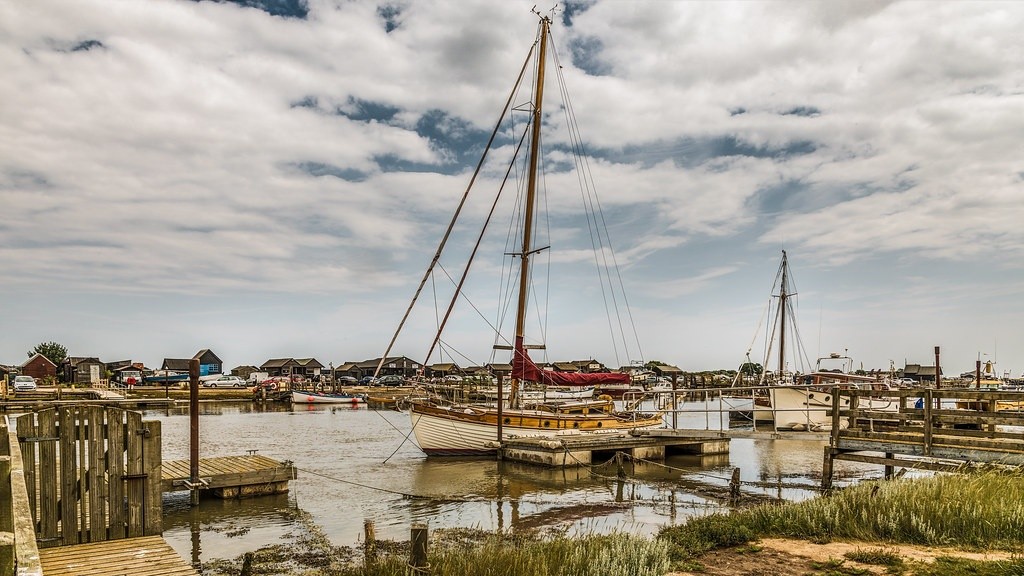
[899,376,919,386]
[311,373,333,386]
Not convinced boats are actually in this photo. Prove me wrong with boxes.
[289,386,367,403]
[144,371,190,382]
[954,359,1024,413]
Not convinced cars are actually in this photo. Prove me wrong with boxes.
[288,374,306,382]
[445,373,495,382]
[336,376,358,387]
[646,375,686,385]
[358,375,378,386]
[12,375,38,394]
[203,375,246,389]
[372,375,404,388]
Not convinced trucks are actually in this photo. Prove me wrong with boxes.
[120,370,143,386]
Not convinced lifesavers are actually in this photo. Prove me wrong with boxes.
[270,383,277,390]
[316,382,323,389]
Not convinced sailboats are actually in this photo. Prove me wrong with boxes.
[360,3,669,460]
[727,249,922,432]
